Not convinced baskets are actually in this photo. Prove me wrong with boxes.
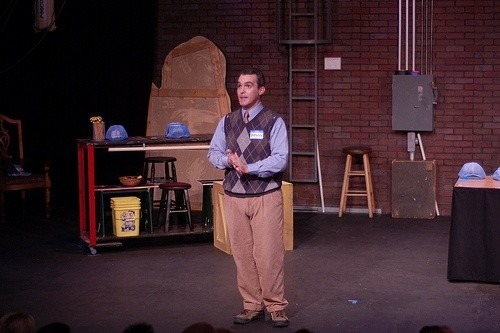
[119,176,142,186]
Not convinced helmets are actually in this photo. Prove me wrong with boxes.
[165,122,190,138]
[491,167,500,180]
[458,163,486,180]
[106,125,128,140]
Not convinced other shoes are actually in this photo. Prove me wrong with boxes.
[270,310,289,327]
[234,309,265,324]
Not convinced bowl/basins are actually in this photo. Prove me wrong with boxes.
[411,71,420,74]
[395,70,410,75]
[119,176,142,186]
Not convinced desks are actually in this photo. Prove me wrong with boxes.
[447,177,499,285]
[76,134,217,249]
[93,181,158,237]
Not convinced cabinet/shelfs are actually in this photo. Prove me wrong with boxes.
[213,181,294,255]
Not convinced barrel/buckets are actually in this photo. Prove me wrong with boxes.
[110,196,143,237]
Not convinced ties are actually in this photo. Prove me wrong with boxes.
[243,111,249,124]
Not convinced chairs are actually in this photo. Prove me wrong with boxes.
[0,114,51,221]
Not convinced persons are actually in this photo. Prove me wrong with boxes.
[419,326,451,333]
[0,312,72,333]
[122,322,309,333]
[207,68,288,327]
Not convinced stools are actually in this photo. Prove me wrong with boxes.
[142,157,176,226]
[338,145,375,218]
[160,182,194,233]
